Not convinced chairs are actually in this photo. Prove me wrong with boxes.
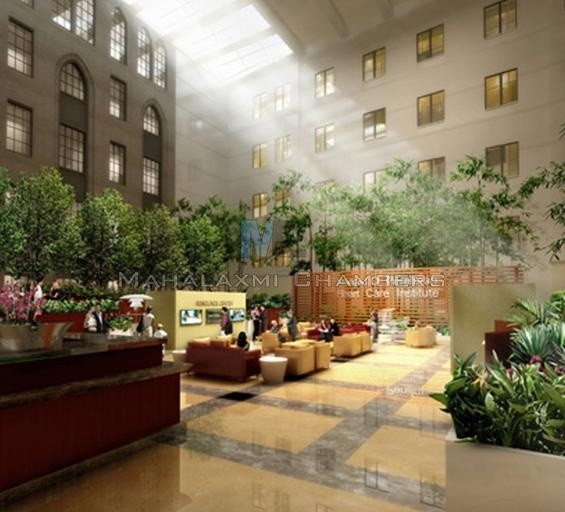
[406,325,436,347]
[187,318,372,383]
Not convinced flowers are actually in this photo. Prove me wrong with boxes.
[430,354,565,455]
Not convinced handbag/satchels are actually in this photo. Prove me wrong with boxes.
[135,316,142,333]
[224,321,232,334]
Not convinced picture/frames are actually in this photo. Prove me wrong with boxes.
[207,308,224,324]
[229,309,246,322]
[180,309,204,326]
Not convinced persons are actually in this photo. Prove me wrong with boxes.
[220,305,379,352]
[34,278,47,300]
[92,302,115,335]
[83,302,98,334]
[154,323,169,356]
[49,281,60,300]
[136,305,157,337]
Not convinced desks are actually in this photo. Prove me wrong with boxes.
[0,336,193,512]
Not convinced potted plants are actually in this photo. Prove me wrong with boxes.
[0,271,144,347]
